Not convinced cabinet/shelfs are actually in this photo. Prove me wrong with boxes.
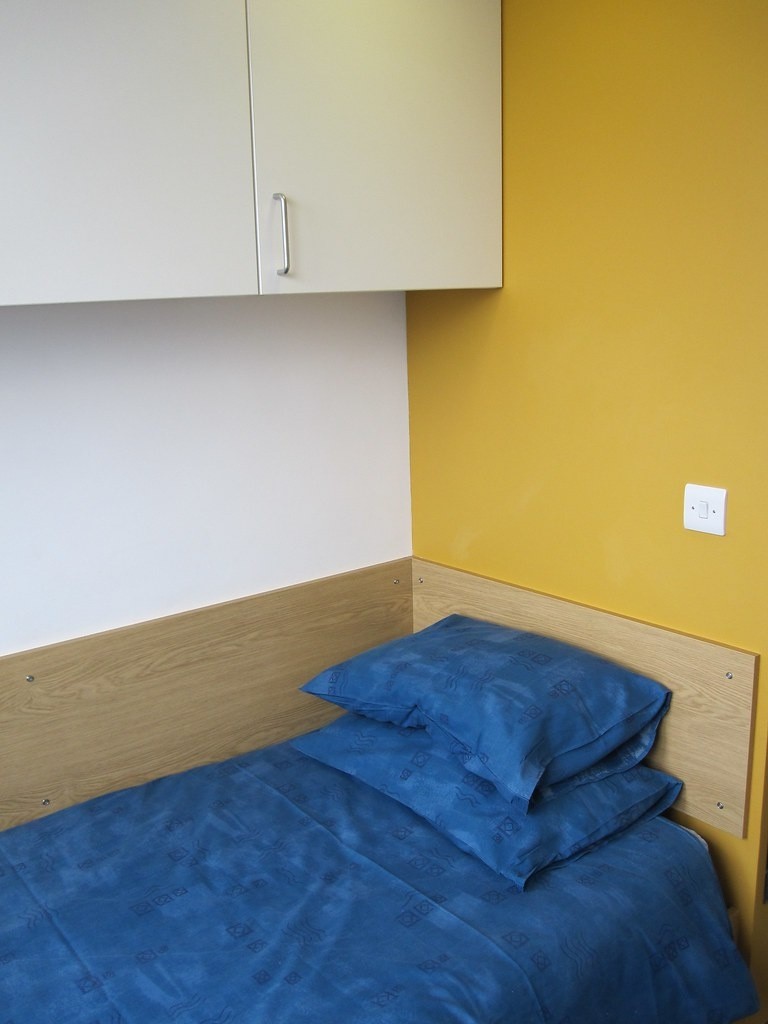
[0,0,504,313]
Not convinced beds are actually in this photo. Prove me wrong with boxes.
[0,707,766,1024]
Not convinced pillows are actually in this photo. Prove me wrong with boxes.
[286,711,684,892]
[299,613,674,816]
